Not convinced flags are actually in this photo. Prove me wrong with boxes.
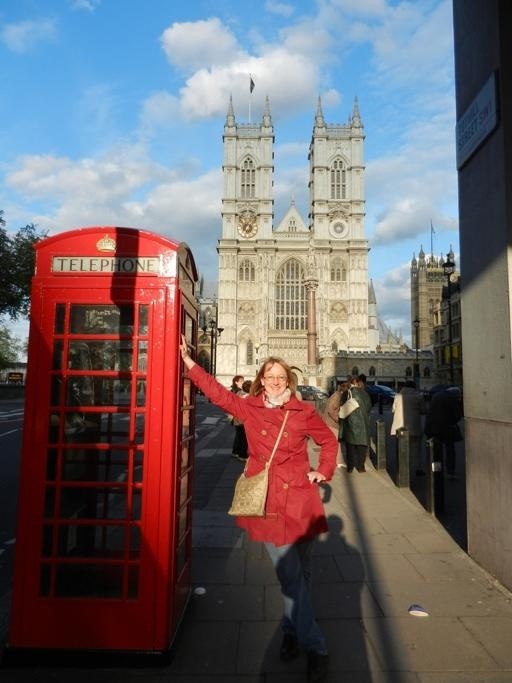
[250,78,255,93]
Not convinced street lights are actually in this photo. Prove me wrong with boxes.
[442,254,455,387]
[202,319,224,402]
[413,316,420,393]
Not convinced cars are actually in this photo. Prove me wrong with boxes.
[370,385,395,404]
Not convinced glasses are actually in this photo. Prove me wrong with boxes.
[262,373,288,381]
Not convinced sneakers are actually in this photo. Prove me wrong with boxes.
[415,469,425,476]
[307,649,329,682]
[447,474,456,479]
[231,453,248,461]
[279,632,300,661]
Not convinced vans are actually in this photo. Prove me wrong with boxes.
[297,386,322,400]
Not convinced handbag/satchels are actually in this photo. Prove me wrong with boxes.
[337,389,359,419]
[226,468,269,518]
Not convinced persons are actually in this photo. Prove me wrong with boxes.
[179,334,335,682]
[390,379,426,475]
[233,379,252,461]
[225,375,244,458]
[321,381,349,468]
[337,375,372,472]
[425,368,463,480]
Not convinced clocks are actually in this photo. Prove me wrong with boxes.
[238,219,257,238]
